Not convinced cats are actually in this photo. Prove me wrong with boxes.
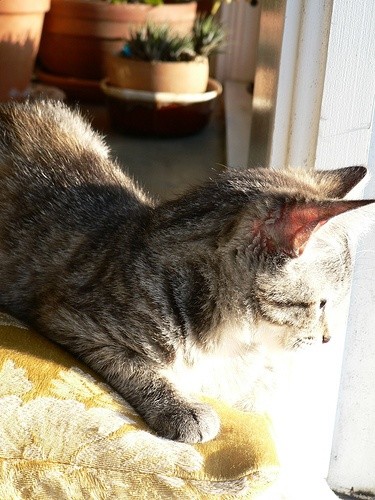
[1,90,375,447]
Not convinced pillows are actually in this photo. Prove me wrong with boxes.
[0,310,274,500]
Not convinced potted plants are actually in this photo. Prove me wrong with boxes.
[32,0,198,96]
[100,16,228,141]
